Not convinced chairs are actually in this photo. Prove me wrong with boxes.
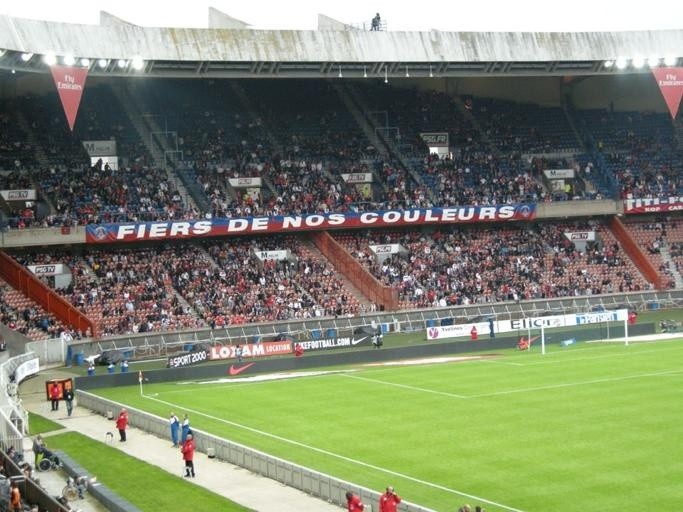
[1,211,683,340]
[0,95,683,230]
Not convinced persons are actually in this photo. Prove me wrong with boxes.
[234,343,243,363]
[32,432,44,471]
[458,504,471,512]
[180,413,190,446]
[470,325,477,340]
[370,334,378,347]
[115,407,128,443]
[0,90,683,339]
[180,434,195,478]
[475,505,484,511]
[87,363,95,375]
[107,361,115,373]
[118,360,128,372]
[0,445,86,511]
[378,483,400,512]
[293,342,302,357]
[169,412,180,447]
[345,490,363,512]
[64,385,74,416]
[40,442,62,467]
[48,380,60,412]
[375,333,383,346]
